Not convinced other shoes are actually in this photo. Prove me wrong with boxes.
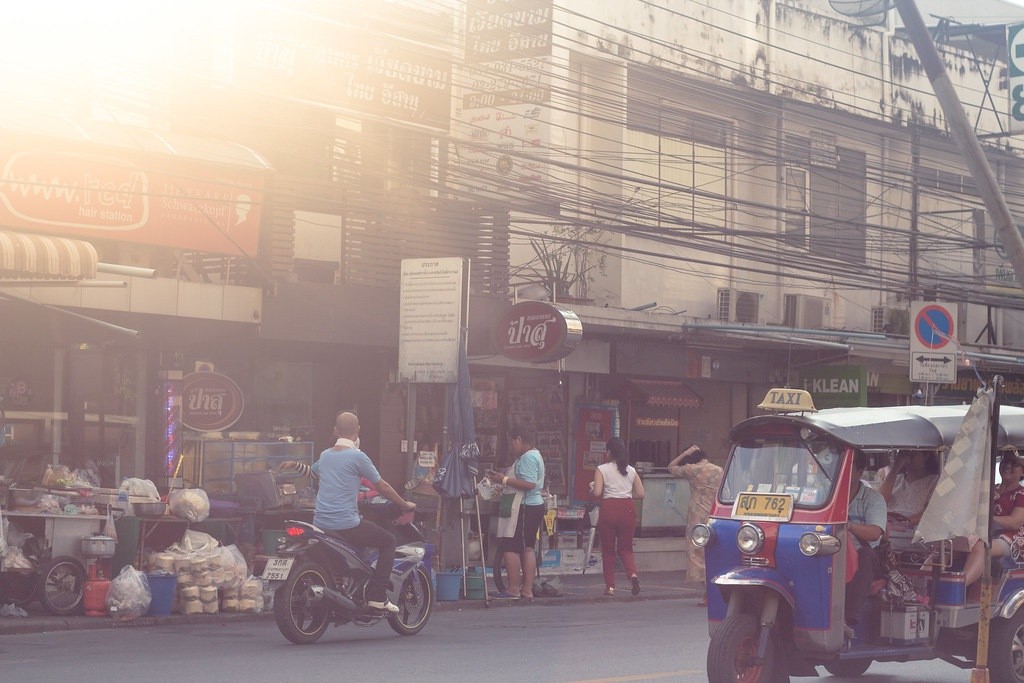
[632,574,641,597]
[604,586,617,595]
[487,590,518,599]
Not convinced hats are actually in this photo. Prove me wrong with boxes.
[1000,456,1024,468]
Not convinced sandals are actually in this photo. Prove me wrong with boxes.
[368,596,399,613]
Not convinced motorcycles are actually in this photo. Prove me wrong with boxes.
[691,386,1023,683]
[262,495,434,645]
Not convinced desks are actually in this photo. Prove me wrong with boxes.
[112,513,245,580]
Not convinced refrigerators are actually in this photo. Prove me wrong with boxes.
[96,487,149,516]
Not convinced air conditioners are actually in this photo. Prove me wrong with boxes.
[718,285,769,329]
[781,293,834,332]
[871,303,911,335]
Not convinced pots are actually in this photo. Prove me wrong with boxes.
[80,532,115,558]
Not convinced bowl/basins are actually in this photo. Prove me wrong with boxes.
[131,501,169,518]
[8,488,44,508]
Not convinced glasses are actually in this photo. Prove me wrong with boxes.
[1000,459,1019,466]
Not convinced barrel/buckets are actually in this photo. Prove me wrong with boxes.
[463,573,486,600]
[139,574,177,616]
[436,571,462,601]
[261,529,288,558]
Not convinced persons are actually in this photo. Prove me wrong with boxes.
[816,448,889,637]
[277,429,384,504]
[584,436,645,597]
[916,449,1024,588]
[877,450,941,551]
[667,445,734,598]
[488,422,545,601]
[310,414,418,614]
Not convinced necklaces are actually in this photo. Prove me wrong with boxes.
[1000,483,1018,490]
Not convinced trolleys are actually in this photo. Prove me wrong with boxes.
[0,486,126,618]
[402,480,556,596]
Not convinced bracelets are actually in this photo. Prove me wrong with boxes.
[895,461,904,467]
[501,476,509,486]
[363,491,368,501]
[684,451,691,456]
